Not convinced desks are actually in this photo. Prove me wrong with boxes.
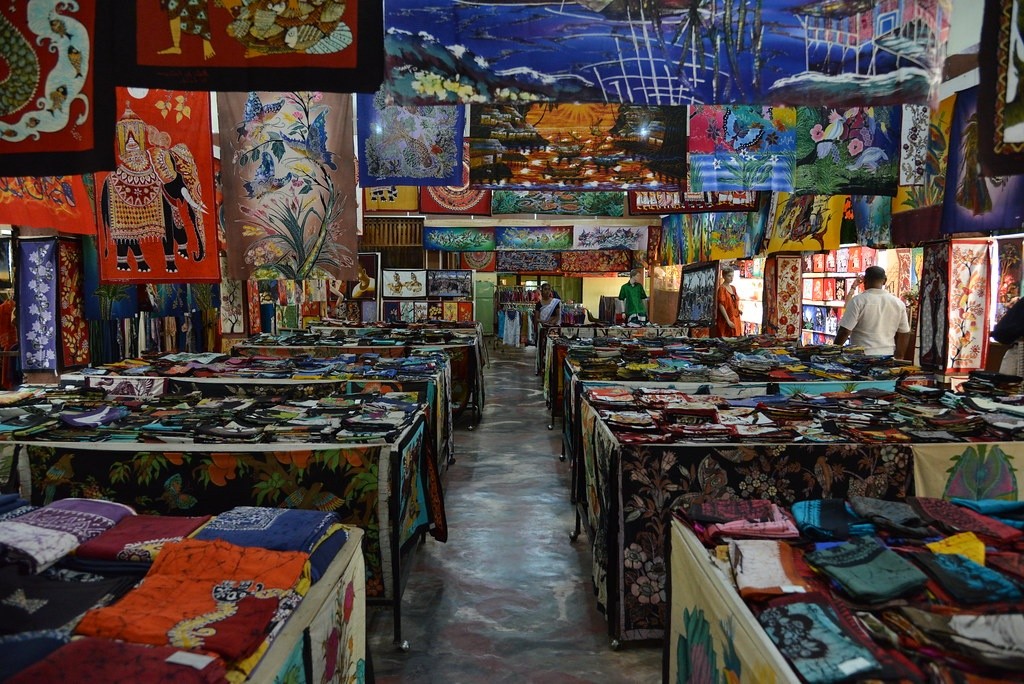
[665,494,1024,683]
[548,323,1022,646]
[0,322,486,684]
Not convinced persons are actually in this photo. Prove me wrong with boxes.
[844,273,865,309]
[990,292,1024,381]
[716,267,743,339]
[533,283,561,376]
[618,269,648,324]
[833,266,911,361]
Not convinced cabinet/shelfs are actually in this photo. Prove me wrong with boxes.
[801,242,861,335]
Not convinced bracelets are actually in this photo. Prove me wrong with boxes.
[850,285,856,291]
